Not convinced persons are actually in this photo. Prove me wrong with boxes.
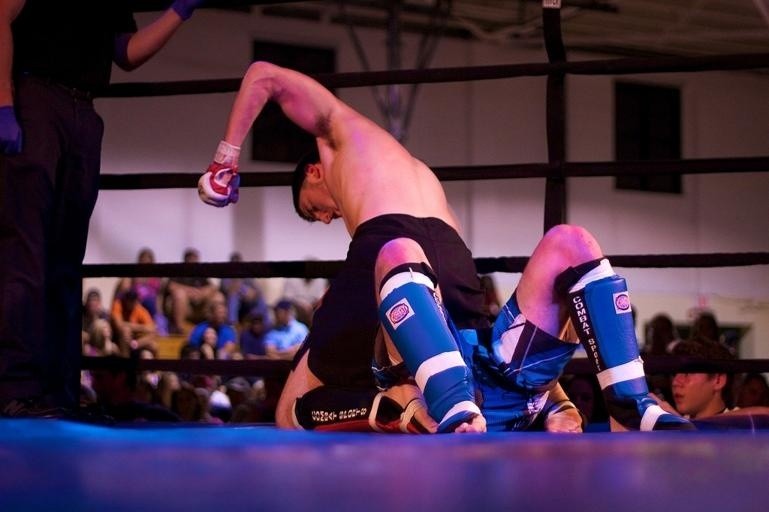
[83,245,328,426]
[197,61,583,432]
[375,226,697,435]
[1,0,191,418]
[644,312,768,418]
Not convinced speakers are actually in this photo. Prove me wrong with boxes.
[613,79,682,192]
[249,34,338,167]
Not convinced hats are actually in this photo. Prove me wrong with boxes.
[667,336,735,375]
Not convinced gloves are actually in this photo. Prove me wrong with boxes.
[196,140,240,208]
[170,0,201,21]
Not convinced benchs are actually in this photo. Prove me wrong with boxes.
[153,334,187,359]
